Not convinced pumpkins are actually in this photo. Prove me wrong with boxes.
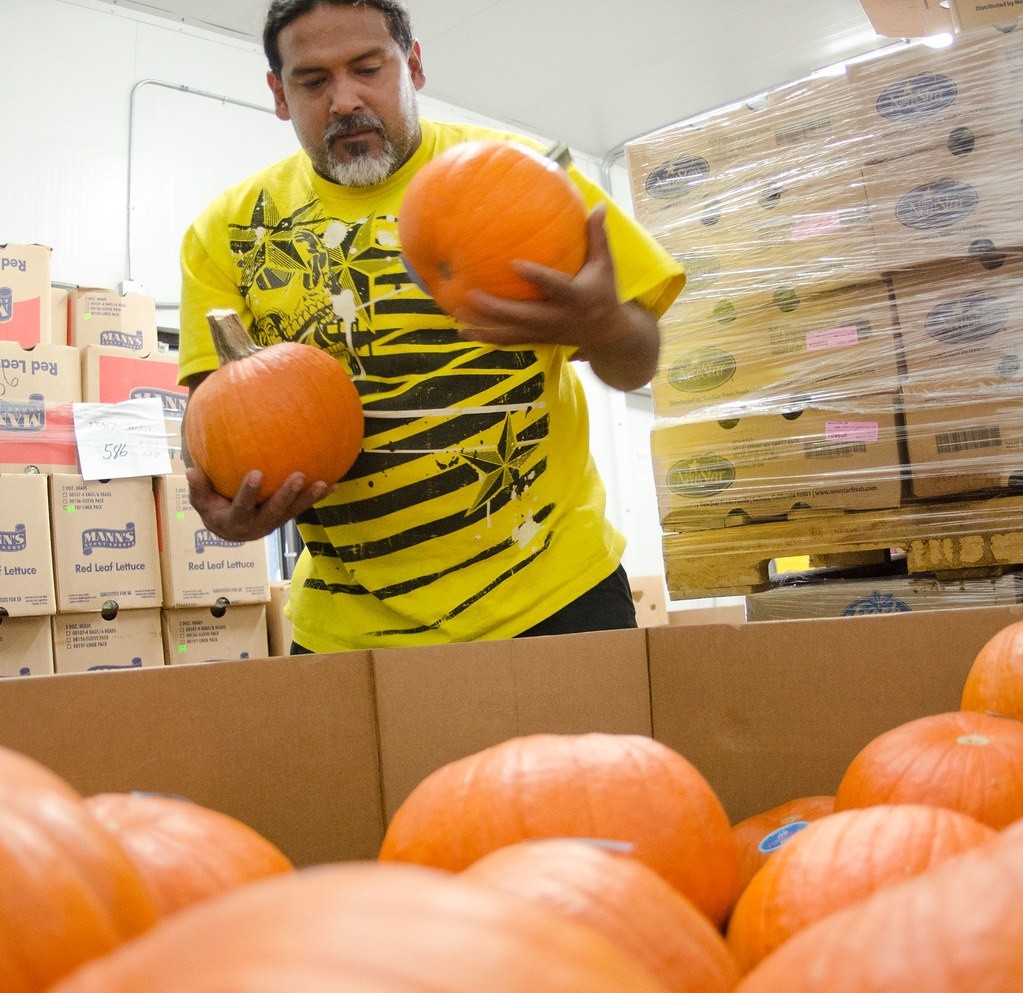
[0,621,1023,993]
[396,143,588,327]
[184,310,364,505]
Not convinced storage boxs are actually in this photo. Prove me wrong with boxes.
[0,243,298,680]
[622,2,1023,627]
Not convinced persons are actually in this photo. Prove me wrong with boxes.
[178,0,684,656]
